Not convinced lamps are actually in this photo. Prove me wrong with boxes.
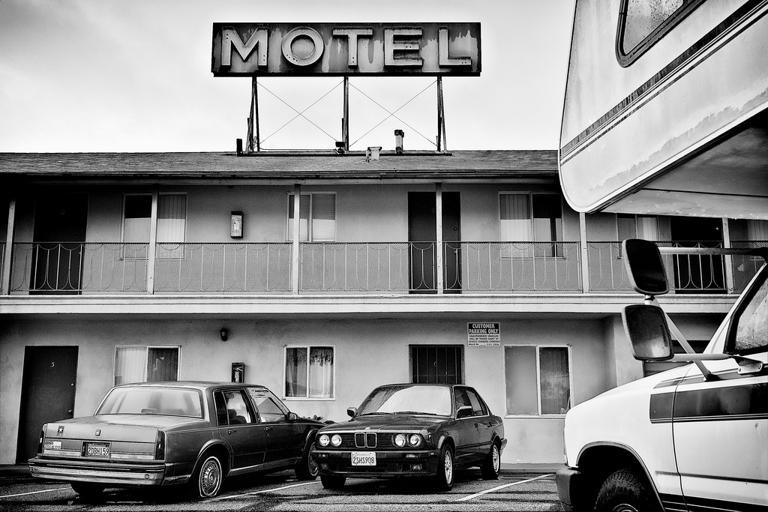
[220,327,229,341]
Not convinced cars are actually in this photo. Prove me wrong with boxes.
[27,379,327,500]
[310,382,507,490]
[554,242,767,511]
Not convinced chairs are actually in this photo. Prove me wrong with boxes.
[218,409,247,425]
